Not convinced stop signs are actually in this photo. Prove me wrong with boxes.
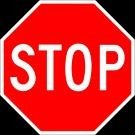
[0,0,135,135]
